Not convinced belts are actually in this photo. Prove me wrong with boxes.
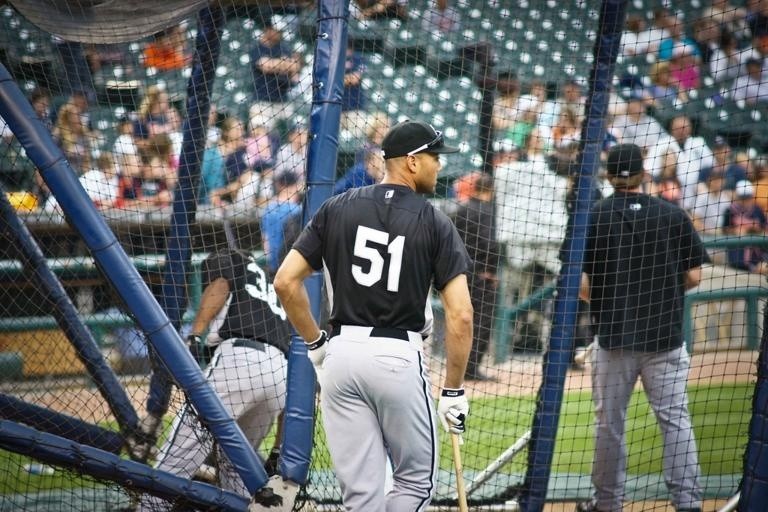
[233,340,264,352]
[330,326,408,340]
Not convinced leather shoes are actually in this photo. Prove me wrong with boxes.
[576,502,595,512]
[467,370,495,381]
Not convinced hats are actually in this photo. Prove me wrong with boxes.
[510,94,537,116]
[673,42,693,57]
[704,132,724,150]
[382,120,460,160]
[735,180,753,196]
[608,144,642,178]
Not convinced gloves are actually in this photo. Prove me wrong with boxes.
[304,330,329,380]
[437,383,469,435]
